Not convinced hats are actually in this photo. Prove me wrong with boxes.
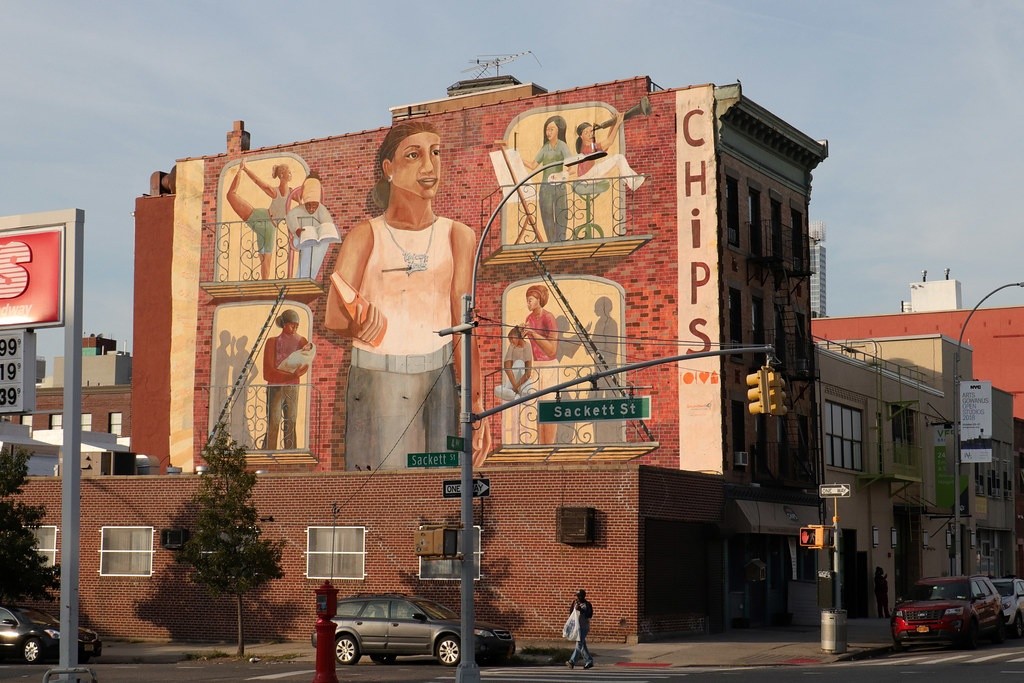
[574,589,586,597]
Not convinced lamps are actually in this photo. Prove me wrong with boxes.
[871,525,879,549]
[946,529,952,549]
[920,529,929,549]
[890,527,897,549]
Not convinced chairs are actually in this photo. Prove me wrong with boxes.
[375,606,385,618]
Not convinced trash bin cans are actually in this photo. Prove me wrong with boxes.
[820,608,848,654]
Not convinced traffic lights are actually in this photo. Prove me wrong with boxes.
[800,524,830,549]
[746,370,768,415]
[767,371,787,416]
[414,521,463,559]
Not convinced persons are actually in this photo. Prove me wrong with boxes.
[1001,569,1017,579]
[565,590,594,670]
[874,566,891,620]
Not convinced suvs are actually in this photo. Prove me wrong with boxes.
[987,577,1024,638]
[890,573,1006,651]
[310,592,516,668]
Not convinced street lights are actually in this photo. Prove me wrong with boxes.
[456,151,609,683]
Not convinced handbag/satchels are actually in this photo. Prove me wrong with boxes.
[562,605,582,641]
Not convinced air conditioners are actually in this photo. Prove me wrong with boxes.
[733,452,749,466]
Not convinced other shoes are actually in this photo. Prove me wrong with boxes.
[584,663,593,670]
[566,660,574,669]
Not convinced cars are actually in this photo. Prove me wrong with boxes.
[0,603,102,665]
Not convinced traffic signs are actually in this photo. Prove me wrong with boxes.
[819,484,851,498]
[442,477,492,498]
